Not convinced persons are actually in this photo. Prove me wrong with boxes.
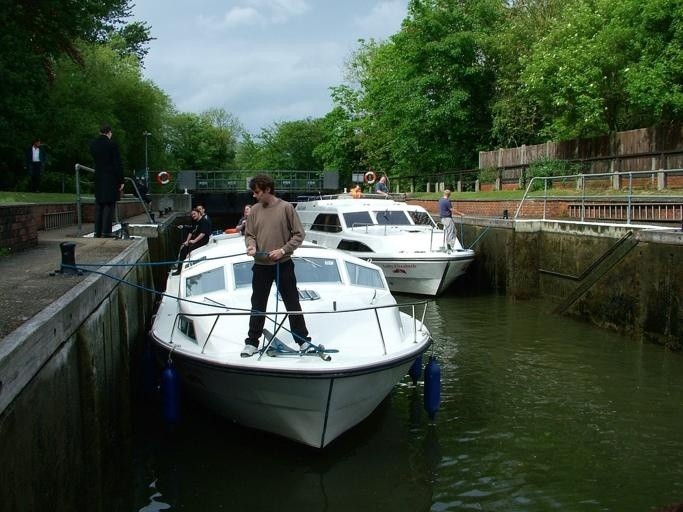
[438,189,466,254]
[240,174,323,358]
[235,205,252,236]
[169,207,209,276]
[373,175,390,195]
[177,205,211,239]
[89,124,125,237]
[25,138,45,193]
[135,176,153,213]
[348,184,365,199]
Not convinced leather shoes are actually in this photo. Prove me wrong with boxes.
[94,232,118,238]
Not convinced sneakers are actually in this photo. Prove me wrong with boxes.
[300,342,318,353]
[172,263,180,276]
[240,344,259,358]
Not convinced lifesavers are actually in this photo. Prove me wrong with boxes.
[364,171,376,184]
[225,229,240,234]
[157,171,171,186]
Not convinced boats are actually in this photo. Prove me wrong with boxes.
[146,228,434,450]
[290,198,476,298]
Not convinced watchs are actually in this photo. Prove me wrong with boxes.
[279,248,285,255]
